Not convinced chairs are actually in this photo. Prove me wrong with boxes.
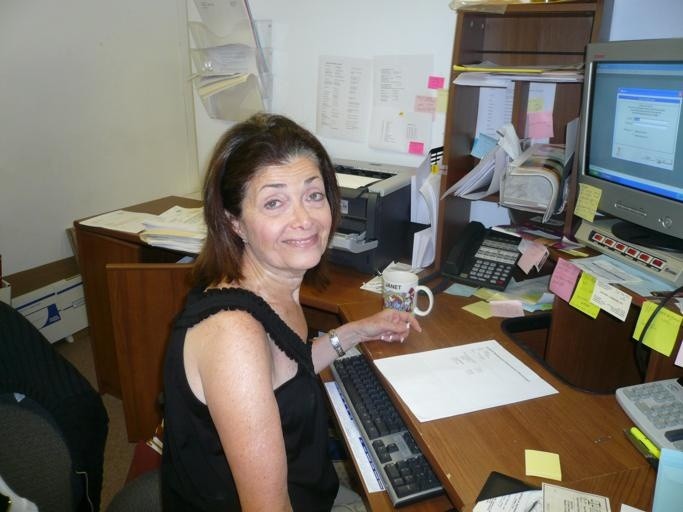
[0,301,163,512]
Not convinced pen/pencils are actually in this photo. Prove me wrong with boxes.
[622,426,661,472]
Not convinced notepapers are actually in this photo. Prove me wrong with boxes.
[525,449,562,482]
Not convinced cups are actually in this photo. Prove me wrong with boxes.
[380,269,434,319]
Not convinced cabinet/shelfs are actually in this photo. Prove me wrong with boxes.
[436,1,611,258]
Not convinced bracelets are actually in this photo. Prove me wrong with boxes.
[328,328,346,356]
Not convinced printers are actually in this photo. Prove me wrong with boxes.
[324,157,419,276]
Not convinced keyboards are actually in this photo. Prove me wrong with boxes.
[329,353,445,507]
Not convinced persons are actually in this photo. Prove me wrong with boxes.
[159,110,423,512]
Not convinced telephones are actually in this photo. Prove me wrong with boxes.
[441,221,524,292]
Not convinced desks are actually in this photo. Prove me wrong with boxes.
[339,258,682,512]
[72,196,412,400]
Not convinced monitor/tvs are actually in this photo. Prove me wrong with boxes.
[577,36,683,254]
